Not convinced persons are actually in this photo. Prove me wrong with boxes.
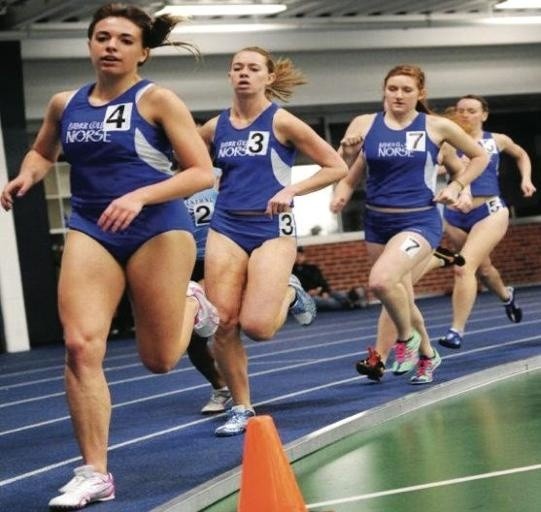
[169,120,233,411]
[336,65,491,385]
[1,4,220,509]
[292,246,369,312]
[438,95,537,349]
[196,47,348,437]
[329,96,465,378]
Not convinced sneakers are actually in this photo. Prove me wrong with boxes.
[391,327,423,376]
[355,350,386,383]
[288,273,318,326]
[433,247,465,266]
[200,386,234,413]
[499,286,522,324]
[215,404,257,437]
[48,465,116,509]
[186,280,220,337]
[410,350,443,384]
[437,329,463,350]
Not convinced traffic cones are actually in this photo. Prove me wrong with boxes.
[236,415,312,512]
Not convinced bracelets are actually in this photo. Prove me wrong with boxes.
[454,178,464,191]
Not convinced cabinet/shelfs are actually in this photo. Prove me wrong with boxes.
[43,160,78,234]
